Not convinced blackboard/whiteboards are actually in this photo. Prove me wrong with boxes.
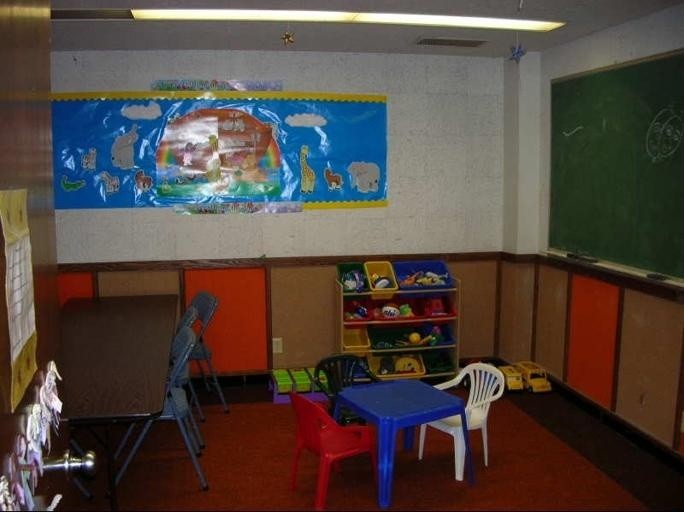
[541,42,684,306]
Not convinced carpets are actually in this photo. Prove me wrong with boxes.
[70,387,652,512]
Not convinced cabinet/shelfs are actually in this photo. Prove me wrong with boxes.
[333,277,461,381]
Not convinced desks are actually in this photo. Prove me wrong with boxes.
[330,379,475,511]
[53,296,178,510]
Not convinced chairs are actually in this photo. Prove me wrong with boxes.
[113,291,227,490]
[418,362,506,481]
[289,356,382,512]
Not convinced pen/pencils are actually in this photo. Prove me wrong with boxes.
[647,273,672,280]
[567,252,600,263]
